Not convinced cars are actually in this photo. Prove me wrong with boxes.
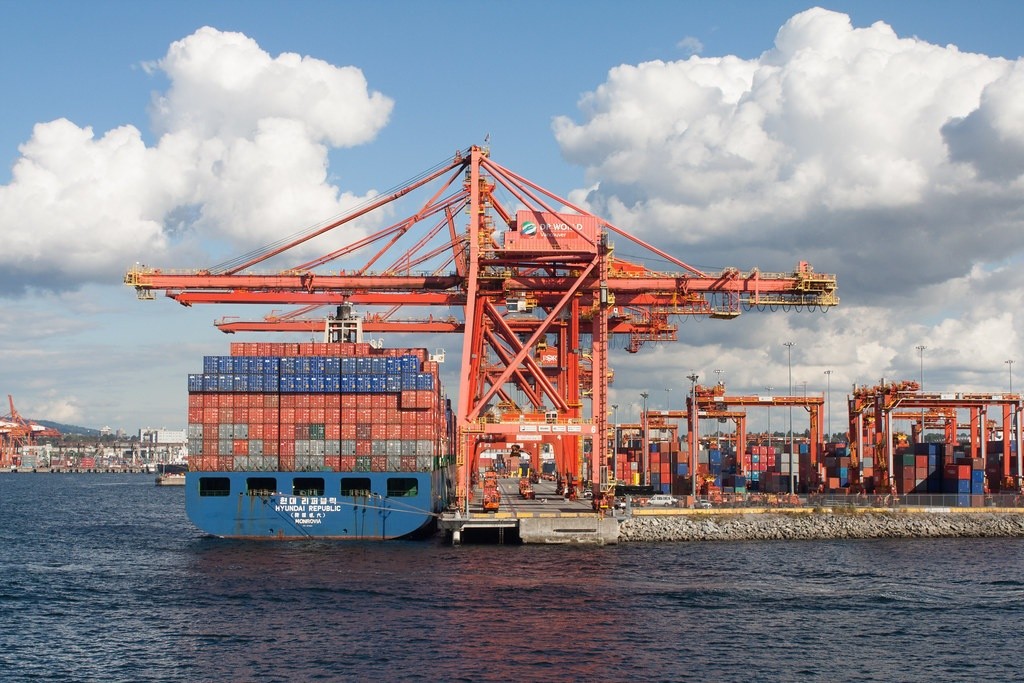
[700,500,712,508]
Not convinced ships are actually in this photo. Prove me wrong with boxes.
[184,295,458,539]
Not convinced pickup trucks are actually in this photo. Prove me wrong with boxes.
[614,499,626,510]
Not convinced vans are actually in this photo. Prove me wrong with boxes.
[647,494,673,507]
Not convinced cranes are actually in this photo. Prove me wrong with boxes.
[120,133,841,515]
[0,394,33,469]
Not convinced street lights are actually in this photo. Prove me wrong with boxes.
[714,369,726,451]
[765,387,777,446]
[915,345,927,443]
[781,342,799,496]
[1004,360,1016,440]
[639,392,650,486]
[824,369,835,443]
[665,388,673,442]
[686,373,701,502]
[611,404,619,484]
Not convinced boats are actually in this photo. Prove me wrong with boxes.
[156,458,189,474]
[155,462,186,485]
